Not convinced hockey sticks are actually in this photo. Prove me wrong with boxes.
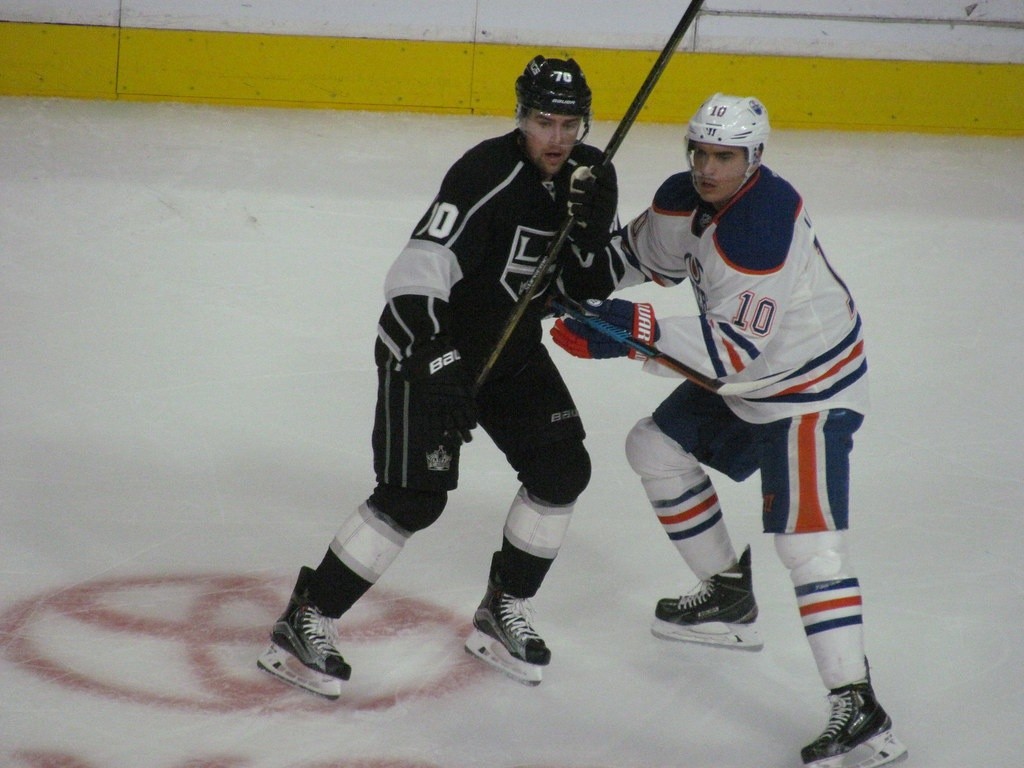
[470,1,704,393]
[545,294,727,396]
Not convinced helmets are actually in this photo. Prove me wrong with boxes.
[515,55,593,131]
[684,92,772,180]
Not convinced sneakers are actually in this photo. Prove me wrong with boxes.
[799,655,908,768]
[465,551,552,687]
[257,566,352,700]
[650,544,765,649]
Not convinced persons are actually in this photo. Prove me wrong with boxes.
[255,54,621,699]
[551,92,907,768]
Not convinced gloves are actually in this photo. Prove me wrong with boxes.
[401,340,491,448]
[549,298,661,363]
